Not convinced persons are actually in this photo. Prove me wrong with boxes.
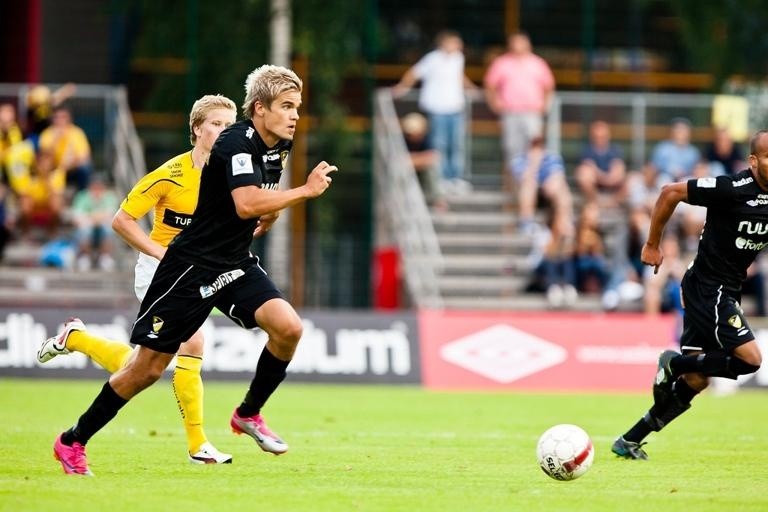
[0,82,119,274]
[54,65,339,478]
[612,131,768,461]
[392,31,480,195]
[37,95,237,464]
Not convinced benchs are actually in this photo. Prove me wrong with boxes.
[0,168,134,311]
[428,191,631,313]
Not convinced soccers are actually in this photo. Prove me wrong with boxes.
[537,424,593,481]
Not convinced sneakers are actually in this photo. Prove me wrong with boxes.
[653,350,681,420]
[187,444,235,465]
[37,315,87,363]
[53,431,96,478]
[609,436,650,461]
[229,406,288,454]
